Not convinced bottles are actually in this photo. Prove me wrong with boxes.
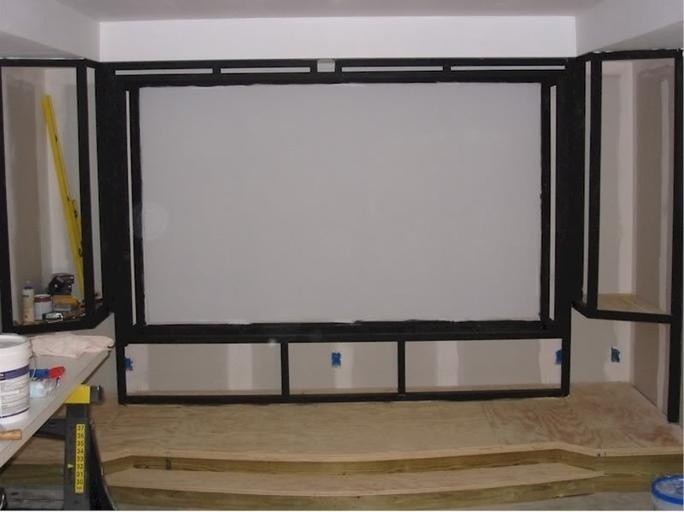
[21,279,36,322]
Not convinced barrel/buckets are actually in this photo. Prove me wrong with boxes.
[0,332,31,425]
[34,294,53,321]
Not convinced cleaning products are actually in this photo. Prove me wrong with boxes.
[22,280,34,322]
